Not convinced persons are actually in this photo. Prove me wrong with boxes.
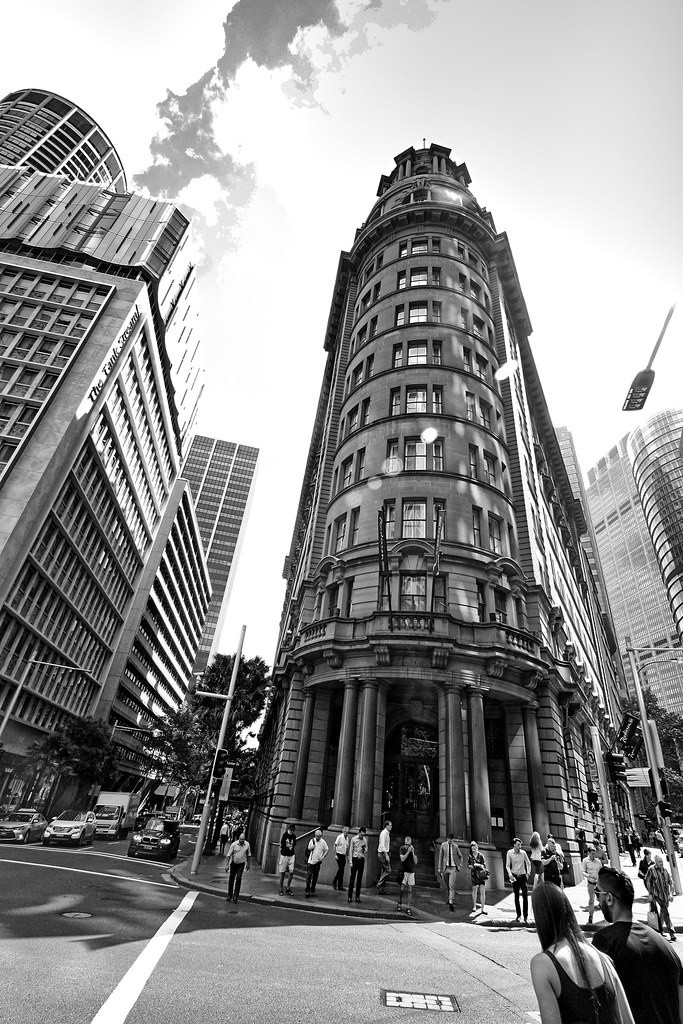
[397,837,417,915]
[530,882,635,1024]
[220,812,247,853]
[438,833,463,912]
[616,825,642,866]
[592,866,683,1024]
[642,828,667,853]
[506,838,531,922]
[306,830,328,896]
[4,792,19,805]
[638,849,676,941]
[348,828,368,903]
[671,828,683,858]
[332,827,350,891]
[581,832,608,923]
[467,841,488,915]
[376,821,392,895]
[279,824,296,895]
[225,834,251,904]
[530,832,564,891]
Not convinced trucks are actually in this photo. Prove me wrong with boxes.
[90,791,141,840]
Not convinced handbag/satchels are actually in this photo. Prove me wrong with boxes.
[477,869,491,880]
[396,867,404,885]
[305,838,315,858]
[647,901,659,929]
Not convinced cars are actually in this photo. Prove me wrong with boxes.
[128,819,182,863]
[0,813,48,844]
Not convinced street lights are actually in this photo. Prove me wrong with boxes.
[87,720,166,811]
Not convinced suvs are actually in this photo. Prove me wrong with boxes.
[43,809,97,847]
[135,806,216,831]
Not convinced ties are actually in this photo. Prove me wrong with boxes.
[449,843,451,867]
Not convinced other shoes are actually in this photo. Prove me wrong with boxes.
[348,897,352,903]
[233,896,238,904]
[376,885,383,893]
[338,888,346,891]
[449,904,454,912]
[482,911,487,915]
[332,883,337,890]
[310,886,315,893]
[305,892,310,898]
[381,892,390,895]
[524,918,528,923]
[517,916,520,922]
[671,935,676,940]
[406,908,412,916]
[472,909,476,912]
[589,916,592,923]
[285,887,294,895]
[397,901,402,911]
[226,896,232,901]
[279,888,284,896]
[356,898,361,903]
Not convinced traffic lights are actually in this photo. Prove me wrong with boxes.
[606,752,627,784]
[658,801,672,818]
[213,749,230,778]
[586,792,599,812]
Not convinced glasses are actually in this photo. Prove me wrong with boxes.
[594,885,619,900]
